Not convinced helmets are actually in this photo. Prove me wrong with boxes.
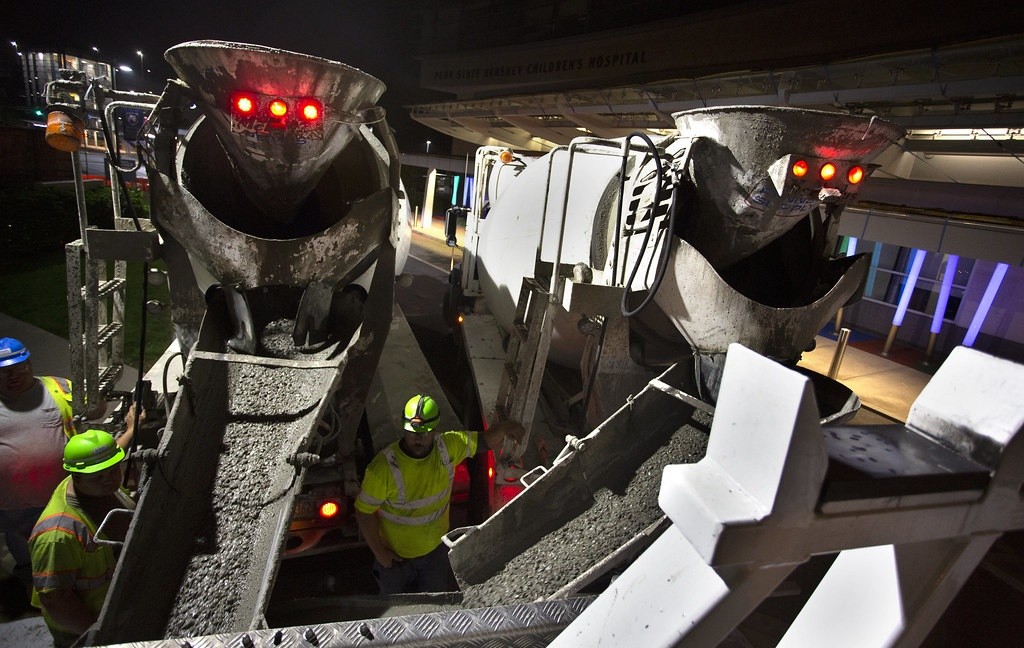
[63,429,125,473]
[403,395,440,432]
[0,337,30,367]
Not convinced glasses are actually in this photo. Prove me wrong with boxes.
[75,466,122,484]
[407,428,435,439]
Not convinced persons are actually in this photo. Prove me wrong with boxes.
[28,402,146,648]
[353,394,526,595]
[0,338,87,602]
